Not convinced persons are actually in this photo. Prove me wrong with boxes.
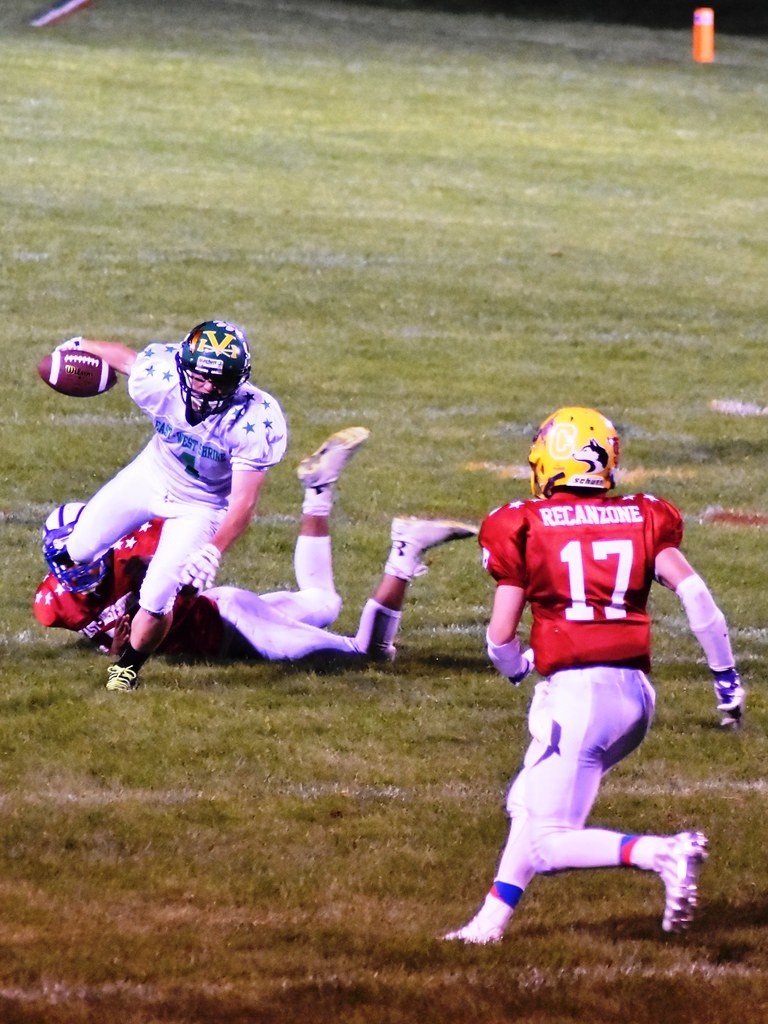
[434,404,745,948]
[41,319,288,697]
[33,424,480,672]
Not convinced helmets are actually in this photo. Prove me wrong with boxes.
[41,502,88,570]
[176,322,252,382]
[527,406,620,493]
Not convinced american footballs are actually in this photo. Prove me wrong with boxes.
[39,350,117,398]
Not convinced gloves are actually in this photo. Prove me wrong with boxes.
[510,649,535,690]
[712,671,744,729]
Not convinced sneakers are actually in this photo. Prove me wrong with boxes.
[298,426,370,489]
[440,916,504,945]
[105,662,140,693]
[390,514,480,551]
[654,830,708,933]
